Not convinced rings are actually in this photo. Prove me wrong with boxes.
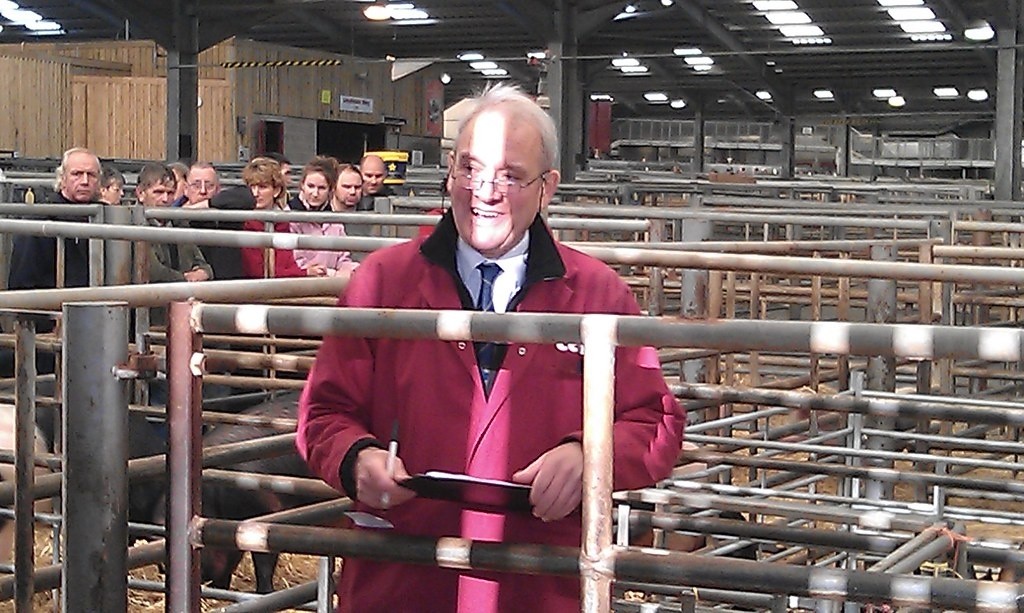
[380,492,389,509]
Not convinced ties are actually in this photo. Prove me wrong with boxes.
[473,262,504,398]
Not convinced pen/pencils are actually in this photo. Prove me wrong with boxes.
[381,420,400,506]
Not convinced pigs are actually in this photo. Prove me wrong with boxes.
[0,371,348,595]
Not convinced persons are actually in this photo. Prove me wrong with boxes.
[7,148,326,375]
[294,85,686,613]
[331,164,367,212]
[359,155,397,211]
[284,160,353,278]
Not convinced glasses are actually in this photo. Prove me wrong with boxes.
[188,180,219,190]
[451,149,552,195]
